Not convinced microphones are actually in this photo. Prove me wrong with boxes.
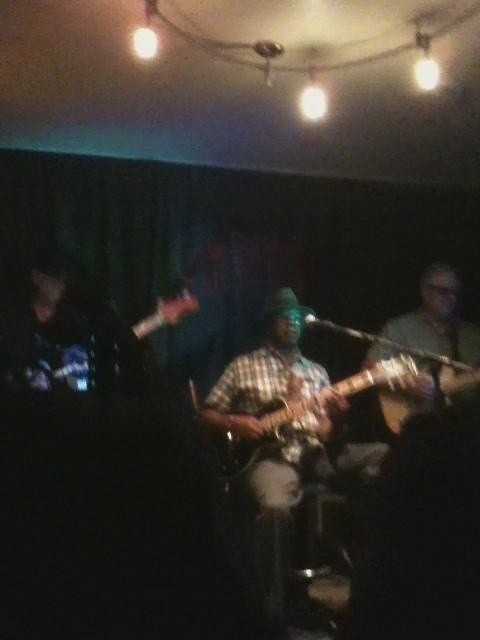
[305,313,341,336]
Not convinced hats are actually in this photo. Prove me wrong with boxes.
[255,286,317,328]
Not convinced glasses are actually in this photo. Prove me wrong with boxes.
[426,283,459,297]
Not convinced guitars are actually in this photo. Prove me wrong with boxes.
[21,287,199,403]
[379,367,478,433]
[205,354,413,482]
